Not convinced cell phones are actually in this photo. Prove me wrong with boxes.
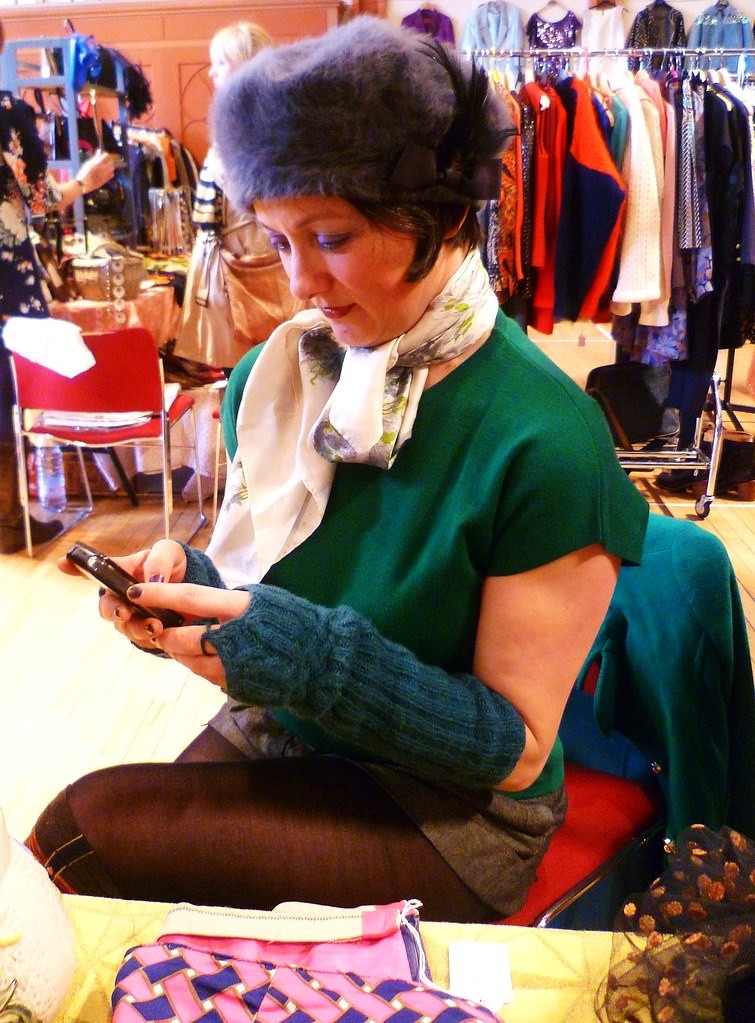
[66,541,184,630]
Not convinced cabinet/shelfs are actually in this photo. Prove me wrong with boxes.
[0,37,137,254]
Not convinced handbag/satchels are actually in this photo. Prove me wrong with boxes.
[220,249,317,343]
[585,364,680,452]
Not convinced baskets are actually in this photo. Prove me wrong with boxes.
[72,243,141,300]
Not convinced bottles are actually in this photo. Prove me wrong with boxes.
[37,444,66,513]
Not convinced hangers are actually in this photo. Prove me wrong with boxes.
[588,0,630,14]
[467,45,752,88]
[653,0,671,19]
[419,1,433,14]
[535,1,568,15]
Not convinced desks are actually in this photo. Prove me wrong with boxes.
[50,286,184,341]
[41,897,665,1022]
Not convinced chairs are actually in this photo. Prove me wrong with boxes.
[7,327,205,558]
[500,513,739,927]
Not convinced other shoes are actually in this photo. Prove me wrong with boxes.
[0,513,64,554]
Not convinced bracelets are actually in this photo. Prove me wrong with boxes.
[77,180,85,197]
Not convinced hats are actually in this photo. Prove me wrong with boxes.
[209,14,508,211]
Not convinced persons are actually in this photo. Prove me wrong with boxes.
[170,23,315,371]
[23,14,650,925]
[0,19,115,554]
